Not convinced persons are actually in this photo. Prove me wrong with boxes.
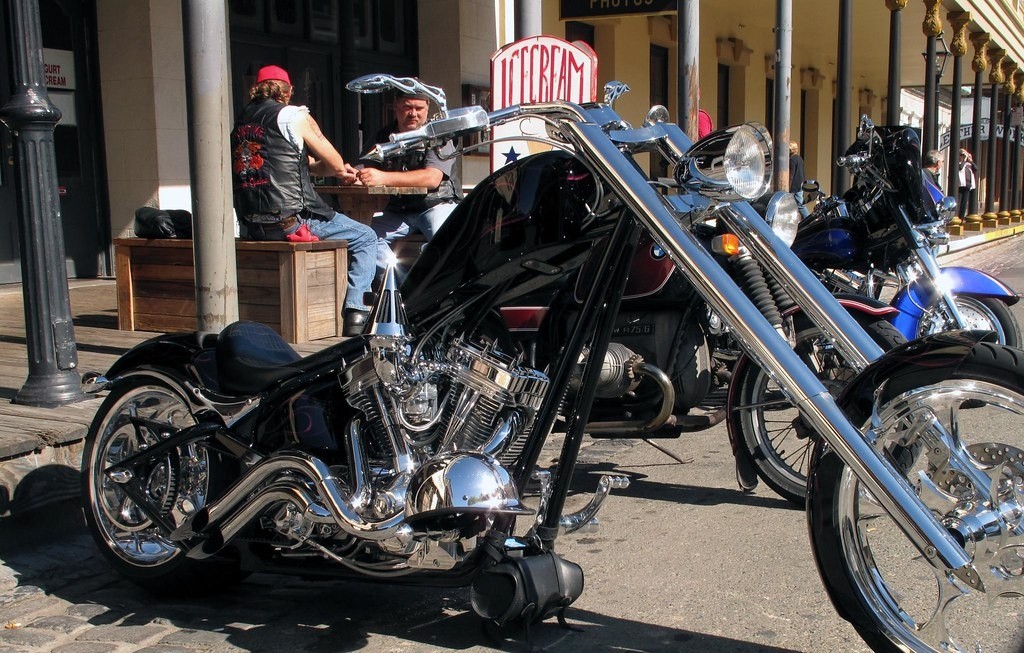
[789,140,810,219]
[341,86,462,293]
[231,65,394,335]
[958,149,979,225]
[922,150,944,258]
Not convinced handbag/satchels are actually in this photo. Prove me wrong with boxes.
[133,207,192,239]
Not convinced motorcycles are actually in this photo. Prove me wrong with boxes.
[77,74,1024,652]
[552,112,1023,509]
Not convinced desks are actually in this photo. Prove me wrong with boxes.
[387,235,429,262]
[310,184,430,225]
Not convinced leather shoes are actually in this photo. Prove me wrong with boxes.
[346,308,370,337]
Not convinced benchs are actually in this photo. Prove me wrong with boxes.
[110,236,350,345]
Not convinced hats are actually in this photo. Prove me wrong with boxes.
[256,65,291,87]
